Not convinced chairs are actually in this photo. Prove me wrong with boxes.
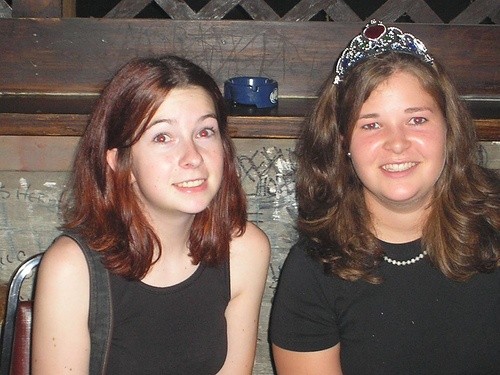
[0,251,45,375]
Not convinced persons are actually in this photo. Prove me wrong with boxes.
[32,52,270,375]
[268,18,500,375]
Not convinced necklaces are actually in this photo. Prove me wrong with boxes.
[380,247,432,265]
